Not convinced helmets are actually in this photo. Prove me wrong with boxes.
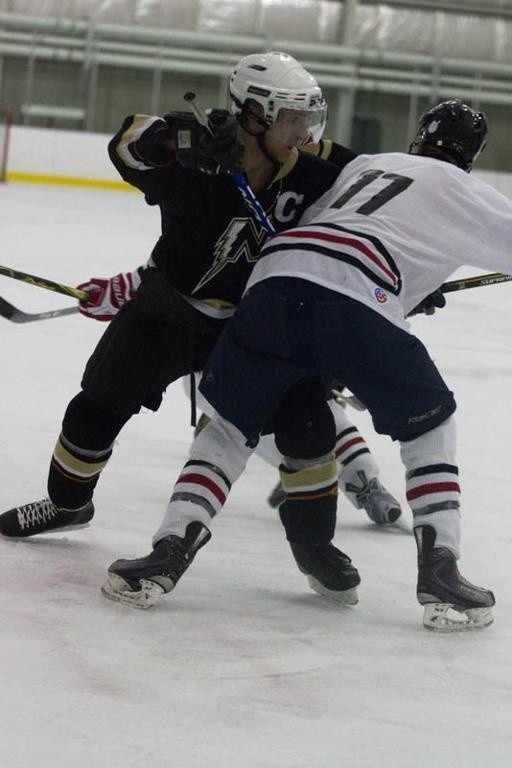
[227,49,329,150]
[408,95,490,176]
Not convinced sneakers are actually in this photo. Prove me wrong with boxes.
[413,543,497,607]
[0,495,95,537]
[106,531,194,594]
[343,469,403,525]
[287,537,361,591]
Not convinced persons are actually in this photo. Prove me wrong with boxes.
[75,123,401,528]
[0,45,451,592]
[105,94,512,609]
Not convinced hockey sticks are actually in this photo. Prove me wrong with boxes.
[184,90,366,414]
[0,296,83,324]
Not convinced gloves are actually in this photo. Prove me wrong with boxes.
[163,106,249,177]
[76,264,146,322]
[403,289,446,317]
